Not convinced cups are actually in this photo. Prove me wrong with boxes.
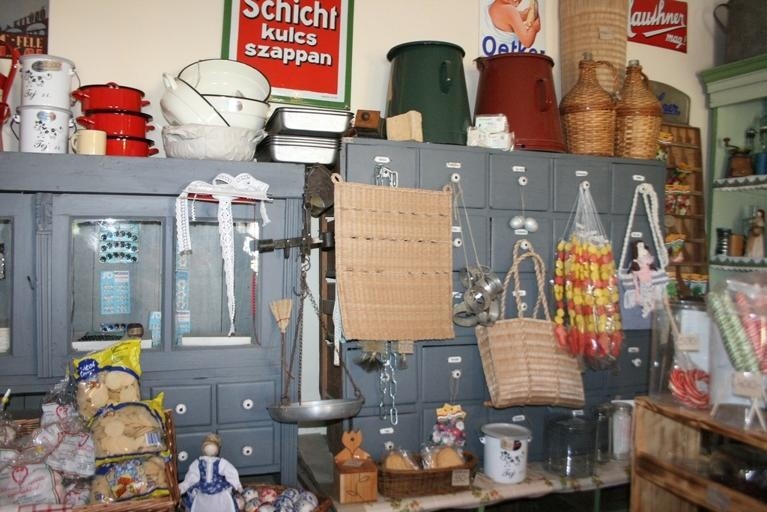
[543,410,595,478]
[71,130,108,156]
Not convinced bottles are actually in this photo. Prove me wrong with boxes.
[744,114,766,154]
[609,402,632,461]
[590,405,608,464]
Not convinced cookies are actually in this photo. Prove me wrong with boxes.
[76,370,170,506]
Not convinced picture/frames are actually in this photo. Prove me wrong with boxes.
[220,1,355,112]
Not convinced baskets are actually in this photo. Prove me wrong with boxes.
[377,450,479,498]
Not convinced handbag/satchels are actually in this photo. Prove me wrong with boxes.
[617,268,670,330]
[475,317,587,409]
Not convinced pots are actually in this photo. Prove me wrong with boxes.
[160,58,271,162]
[71,82,150,112]
[106,137,158,156]
[75,109,155,137]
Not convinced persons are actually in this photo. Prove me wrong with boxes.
[744,208,766,258]
[174,432,244,512]
[488,1,540,55]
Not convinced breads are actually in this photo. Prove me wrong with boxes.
[383,446,463,470]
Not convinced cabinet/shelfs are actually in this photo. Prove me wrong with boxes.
[698,52,767,294]
[318,138,667,467]
[629,395,767,511]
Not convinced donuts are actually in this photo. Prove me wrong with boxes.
[668,367,696,407]
[684,370,710,409]
